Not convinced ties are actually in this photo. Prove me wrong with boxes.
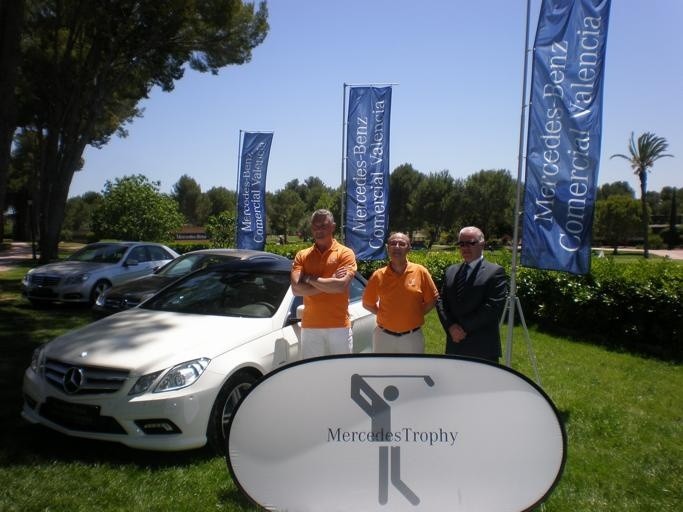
[456,262,467,303]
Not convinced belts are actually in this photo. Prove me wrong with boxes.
[378,325,420,336]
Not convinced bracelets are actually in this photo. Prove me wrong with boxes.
[303,273,312,285]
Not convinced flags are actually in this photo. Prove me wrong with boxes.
[344,84,393,262]
[519,1,614,276]
[236,131,273,251]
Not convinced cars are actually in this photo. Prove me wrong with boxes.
[20,242,180,304]
[20,261,375,452]
[93,248,291,316]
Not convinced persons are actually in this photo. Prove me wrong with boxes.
[360,232,440,356]
[287,207,357,359]
[436,227,508,366]
